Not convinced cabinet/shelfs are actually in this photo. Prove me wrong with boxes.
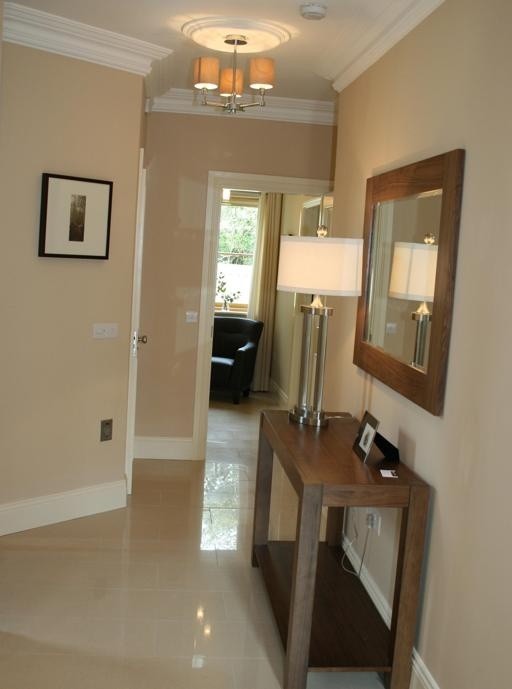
[248,407,438,689]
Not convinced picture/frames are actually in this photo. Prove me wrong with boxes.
[36,171,115,260]
[351,410,400,465]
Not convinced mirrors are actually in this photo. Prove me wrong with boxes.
[349,143,473,419]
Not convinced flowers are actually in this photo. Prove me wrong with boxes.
[214,271,243,310]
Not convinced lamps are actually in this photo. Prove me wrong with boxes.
[175,17,296,117]
[385,231,439,374]
[272,225,365,429]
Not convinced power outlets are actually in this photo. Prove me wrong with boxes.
[365,508,382,537]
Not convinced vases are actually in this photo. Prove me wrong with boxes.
[221,300,230,312]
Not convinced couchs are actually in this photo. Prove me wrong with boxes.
[207,312,264,403]
